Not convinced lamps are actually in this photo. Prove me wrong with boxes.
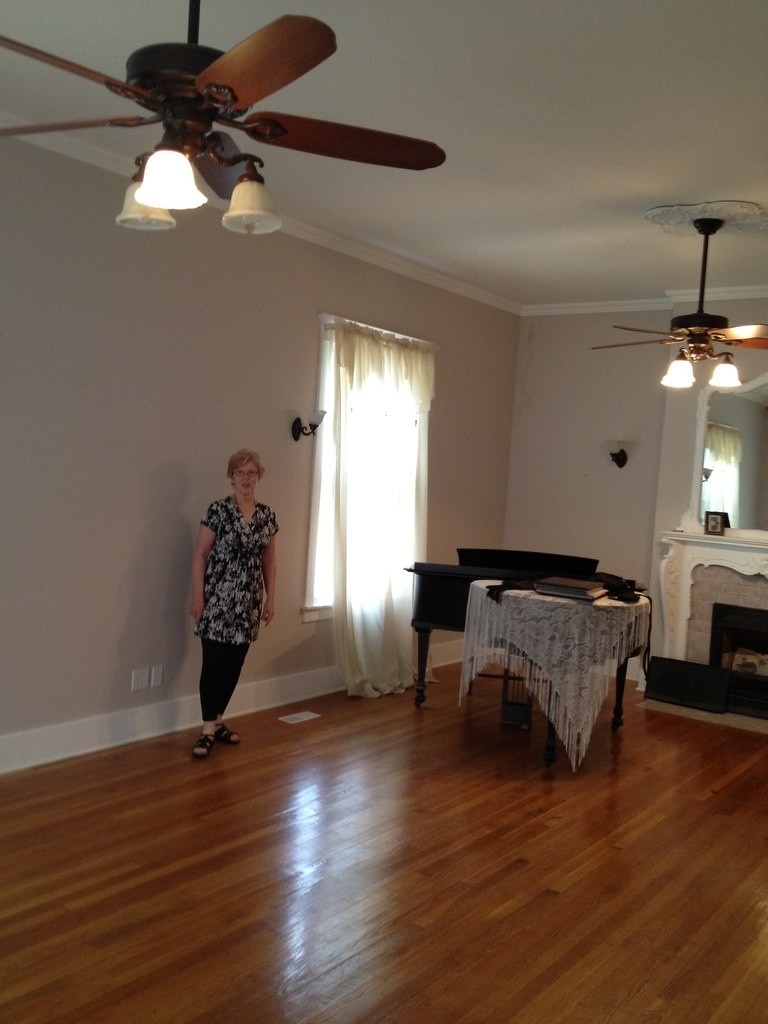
[709,363,742,387]
[221,182,283,234]
[115,182,176,231]
[291,409,327,441]
[134,150,207,209]
[660,360,696,388]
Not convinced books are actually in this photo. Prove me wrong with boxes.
[533,575,608,600]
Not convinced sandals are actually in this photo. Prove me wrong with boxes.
[214,722,241,745]
[192,734,215,759]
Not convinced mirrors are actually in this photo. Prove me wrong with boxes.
[698,381,767,533]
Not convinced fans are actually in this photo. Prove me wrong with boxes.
[589,218,768,363]
[0,1,445,200]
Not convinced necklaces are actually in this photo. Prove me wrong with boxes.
[233,494,257,506]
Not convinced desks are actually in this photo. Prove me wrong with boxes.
[462,579,653,767]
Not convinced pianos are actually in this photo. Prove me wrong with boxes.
[402,545,653,722]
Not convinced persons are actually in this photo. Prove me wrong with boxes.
[190,449,280,759]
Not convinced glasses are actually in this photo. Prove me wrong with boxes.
[231,470,259,477]
